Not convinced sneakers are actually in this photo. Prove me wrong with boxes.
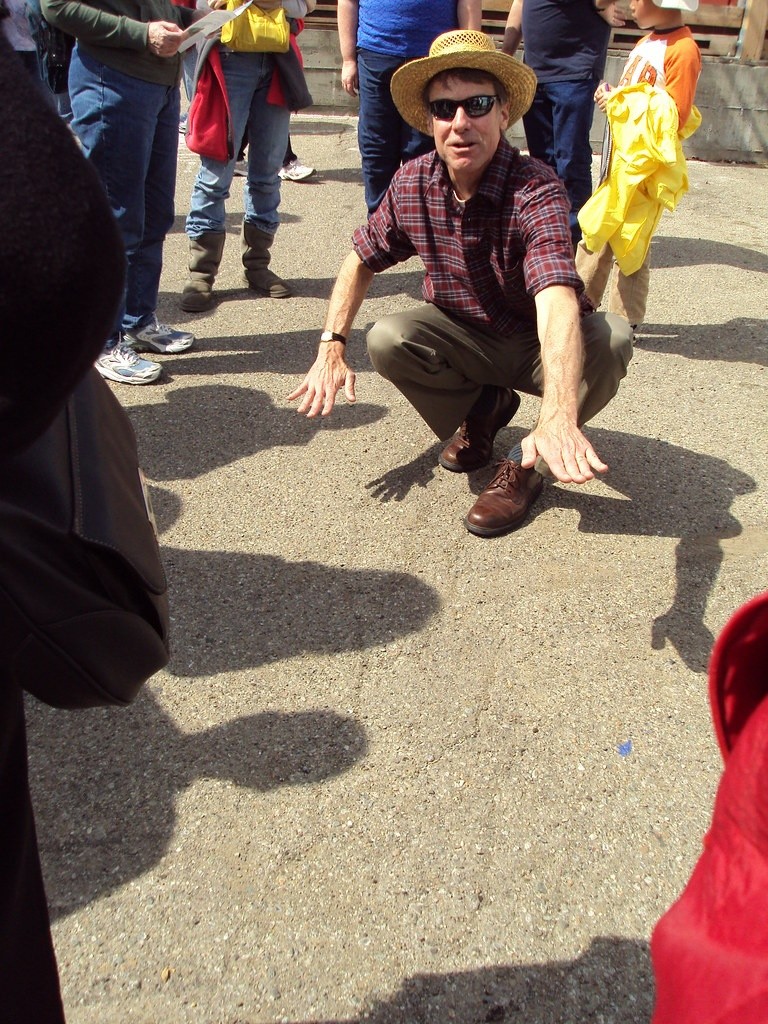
[124,313,194,352]
[93,345,164,385]
[231,161,248,178]
[278,157,317,180]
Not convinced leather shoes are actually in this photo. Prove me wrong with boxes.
[436,386,522,474]
[464,455,543,536]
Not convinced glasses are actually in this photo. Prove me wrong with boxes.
[427,93,498,121]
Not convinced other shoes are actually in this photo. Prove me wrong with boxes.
[179,122,187,133]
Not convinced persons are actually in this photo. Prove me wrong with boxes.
[569,0,702,329]
[501,1,627,258]
[0,0,316,186]
[651,693,768,1024]
[336,0,481,222]
[40,0,211,387]
[178,0,317,311]
[1,36,129,1024]
[285,29,634,539]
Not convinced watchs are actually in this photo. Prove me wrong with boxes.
[320,331,347,346]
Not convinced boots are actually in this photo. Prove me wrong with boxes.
[238,218,292,298]
[180,229,227,312]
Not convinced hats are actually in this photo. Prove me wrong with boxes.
[654,1,700,12]
[390,30,538,138]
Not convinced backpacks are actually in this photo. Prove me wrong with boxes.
[24,0,76,93]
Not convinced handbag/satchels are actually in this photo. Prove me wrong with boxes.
[0,363,171,711]
[219,0,291,54]
